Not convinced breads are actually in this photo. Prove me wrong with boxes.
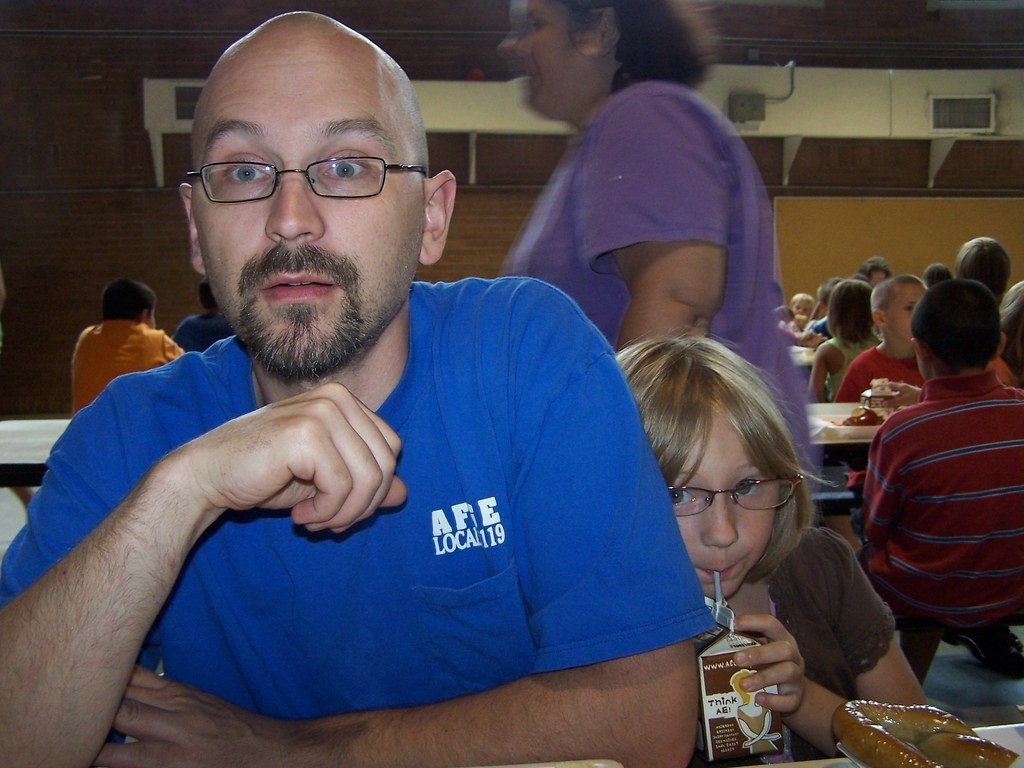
[832,700,1019,768]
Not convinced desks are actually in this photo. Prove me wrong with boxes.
[0,417,71,488]
[786,342,911,501]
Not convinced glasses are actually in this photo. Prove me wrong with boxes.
[187,156,427,204]
[668,473,804,517]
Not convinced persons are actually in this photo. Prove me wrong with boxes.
[497,0,816,481]
[72,277,186,417]
[169,278,236,353]
[777,235,1024,685]
[0,11,717,768]
[613,325,930,768]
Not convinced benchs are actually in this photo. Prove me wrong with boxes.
[885,606,1024,688]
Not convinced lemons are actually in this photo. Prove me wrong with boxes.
[851,406,869,416]
